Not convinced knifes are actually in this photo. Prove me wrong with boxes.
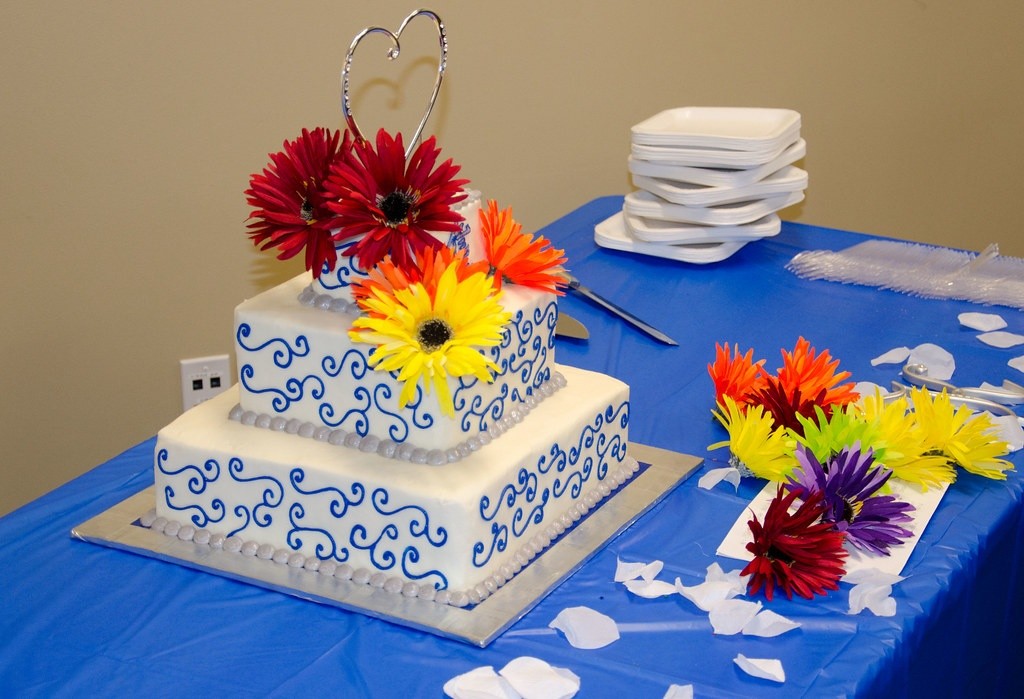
[555,312,590,340]
[556,265,677,345]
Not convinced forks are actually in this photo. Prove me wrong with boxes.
[784,239,1024,312]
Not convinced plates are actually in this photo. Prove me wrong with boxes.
[593,105,808,265]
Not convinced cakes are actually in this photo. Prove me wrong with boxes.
[137,128,641,604]
